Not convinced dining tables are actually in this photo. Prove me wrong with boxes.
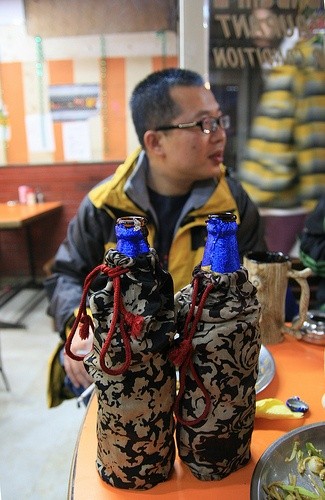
[69,322,325,500]
[0,202,63,289]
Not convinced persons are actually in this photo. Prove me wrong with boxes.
[45,68,269,409]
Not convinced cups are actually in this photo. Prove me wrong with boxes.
[244,251,311,343]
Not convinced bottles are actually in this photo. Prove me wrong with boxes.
[87,216,176,490]
[176,213,262,482]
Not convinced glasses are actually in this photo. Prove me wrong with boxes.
[154,113,231,134]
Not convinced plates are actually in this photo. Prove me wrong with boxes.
[177,340,275,394]
[250,421,325,500]
